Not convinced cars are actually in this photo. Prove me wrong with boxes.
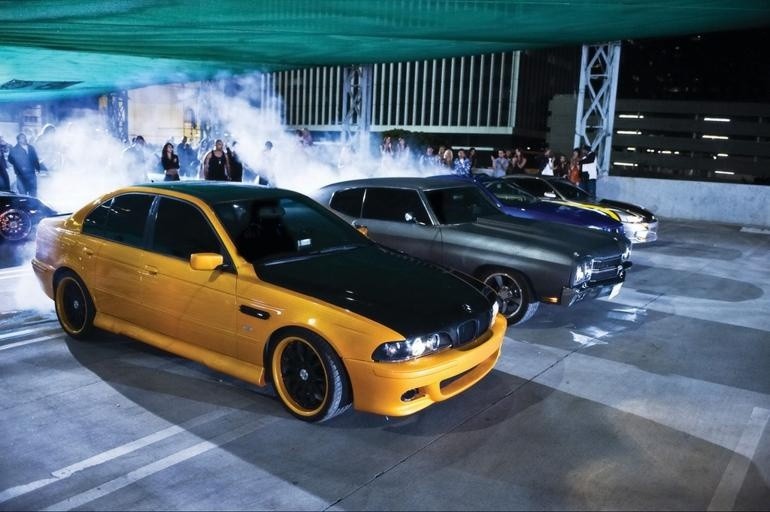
[427,174,628,245]
[487,171,659,245]
[494,150,545,174]
[303,174,634,329]
[0,190,59,244]
[29,174,508,423]
